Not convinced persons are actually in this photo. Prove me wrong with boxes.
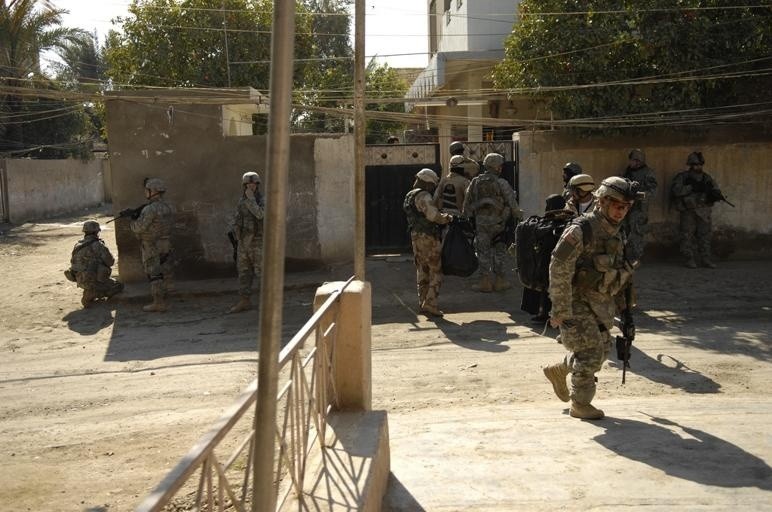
[404,169,453,317]
[564,163,583,195]
[129,179,173,312]
[544,176,639,420]
[227,172,266,313]
[70,221,124,308]
[563,174,598,217]
[434,156,472,239]
[623,149,658,264]
[464,155,525,293]
[448,142,483,179]
[386,136,401,144]
[671,152,724,268]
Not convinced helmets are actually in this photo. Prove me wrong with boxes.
[685,152,705,165]
[82,221,101,233]
[567,174,594,194]
[483,153,503,172]
[563,162,582,177]
[448,155,465,169]
[145,179,166,192]
[628,149,646,165]
[241,172,261,184]
[416,168,439,188]
[546,194,565,212]
[590,177,638,205]
[449,142,465,155]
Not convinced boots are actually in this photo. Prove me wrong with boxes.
[700,261,717,268]
[228,296,252,313]
[470,276,493,292]
[143,301,167,313]
[569,396,604,419]
[544,363,570,402]
[491,277,513,291]
[685,260,696,268]
[421,300,444,316]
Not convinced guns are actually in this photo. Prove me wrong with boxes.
[106,205,146,225]
[617,284,635,385]
[225,232,240,265]
[704,185,737,210]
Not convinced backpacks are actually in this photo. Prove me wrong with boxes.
[516,212,628,294]
